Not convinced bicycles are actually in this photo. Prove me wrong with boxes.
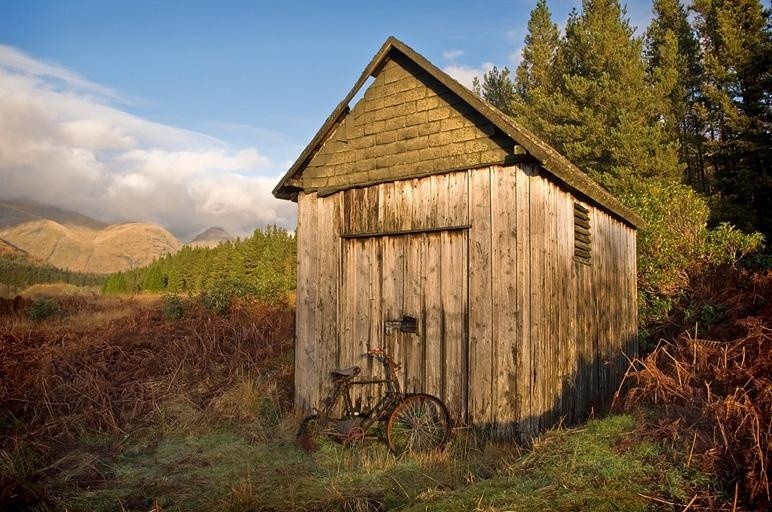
[291,350,451,454]
